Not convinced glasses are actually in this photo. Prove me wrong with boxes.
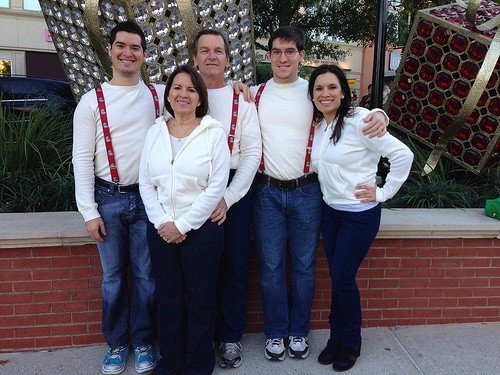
[270,48,303,57]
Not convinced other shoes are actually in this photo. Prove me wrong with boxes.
[317,339,336,364]
[332,349,360,371]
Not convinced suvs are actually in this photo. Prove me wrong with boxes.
[0,72,78,125]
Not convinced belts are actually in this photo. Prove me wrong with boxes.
[95,176,142,192]
[259,171,317,191]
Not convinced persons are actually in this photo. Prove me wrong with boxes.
[307,64,414,371]
[248,26,390,362]
[193,30,262,368]
[72,22,257,375]
[139,65,230,375]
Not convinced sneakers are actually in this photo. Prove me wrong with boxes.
[288,335,311,358]
[216,340,243,368]
[103,345,129,375]
[265,337,287,363]
[133,344,157,374]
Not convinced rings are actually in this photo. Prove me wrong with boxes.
[166,239,167,241]
